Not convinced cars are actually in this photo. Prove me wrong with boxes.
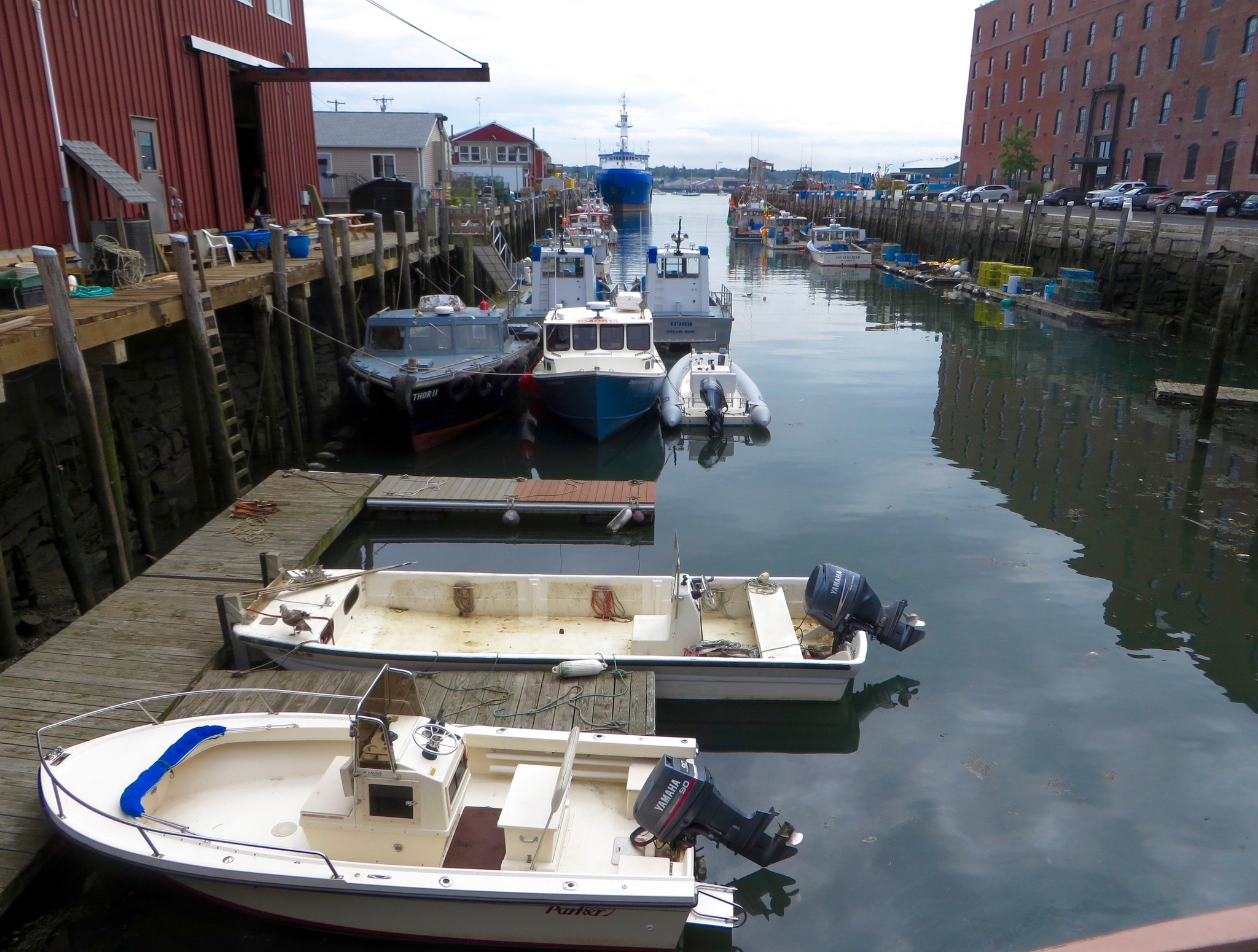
[845,185,864,191]
[961,184,1012,202]
[1042,187,1088,206]
[1145,189,1258,219]
[788,180,807,194]
[937,185,971,202]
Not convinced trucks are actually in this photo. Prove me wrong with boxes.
[905,182,958,200]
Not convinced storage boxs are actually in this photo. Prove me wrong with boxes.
[0,268,48,309]
[563,179,574,188]
[977,261,1104,311]
[863,237,919,267]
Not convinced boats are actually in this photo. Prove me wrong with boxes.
[529,291,668,420]
[800,196,827,240]
[505,137,619,330]
[655,347,773,435]
[716,190,728,196]
[805,208,872,268]
[652,192,667,196]
[231,562,928,705]
[344,293,544,455]
[671,191,678,195]
[34,661,804,952]
[727,131,782,243]
[759,211,808,250]
[593,91,655,213]
[623,211,735,345]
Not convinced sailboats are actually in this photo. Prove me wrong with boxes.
[681,172,701,196]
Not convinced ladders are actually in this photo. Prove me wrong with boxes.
[190,235,251,510]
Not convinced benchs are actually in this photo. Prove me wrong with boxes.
[299,756,356,858]
[690,373,736,407]
[746,585,804,659]
[497,726,580,863]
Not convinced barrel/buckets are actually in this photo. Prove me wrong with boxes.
[959,261,969,272]
[749,222,754,229]
[768,226,775,238]
[1007,275,1021,294]
[14,263,39,279]
[286,235,309,258]
[823,245,832,252]
[784,237,788,244]
[776,231,784,245]
[542,235,550,247]
[881,250,919,266]
[748,226,751,231]
[297,230,320,245]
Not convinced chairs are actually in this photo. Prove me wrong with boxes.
[201,229,235,267]
[305,183,348,257]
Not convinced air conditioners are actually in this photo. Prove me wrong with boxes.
[481,159,491,165]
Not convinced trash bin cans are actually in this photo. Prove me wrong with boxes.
[285,235,309,259]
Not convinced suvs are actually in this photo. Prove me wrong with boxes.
[1101,185,1173,211]
[1084,179,1148,209]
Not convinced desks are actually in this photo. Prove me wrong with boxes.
[325,213,374,241]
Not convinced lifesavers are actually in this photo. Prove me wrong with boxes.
[783,227,789,232]
